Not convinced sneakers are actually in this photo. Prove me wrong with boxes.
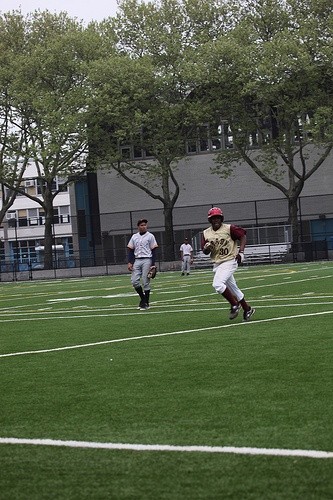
[230,302,242,320]
[242,305,255,321]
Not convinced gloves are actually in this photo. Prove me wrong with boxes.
[203,242,213,254]
[235,250,244,266]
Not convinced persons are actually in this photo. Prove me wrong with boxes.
[201,207,256,321]
[127,219,158,310]
[180,238,193,276]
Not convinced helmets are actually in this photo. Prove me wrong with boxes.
[208,207,224,222]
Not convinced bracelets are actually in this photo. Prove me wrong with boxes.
[239,250,244,253]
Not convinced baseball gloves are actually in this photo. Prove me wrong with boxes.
[146,266,158,279]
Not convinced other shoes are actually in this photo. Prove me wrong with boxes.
[182,273,184,275]
[137,301,151,310]
[187,273,189,275]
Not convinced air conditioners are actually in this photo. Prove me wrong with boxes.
[6,213,15,220]
[24,180,34,187]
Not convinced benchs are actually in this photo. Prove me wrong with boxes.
[191,242,292,270]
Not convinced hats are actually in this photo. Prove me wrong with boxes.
[184,237,188,240]
[137,219,147,226]
